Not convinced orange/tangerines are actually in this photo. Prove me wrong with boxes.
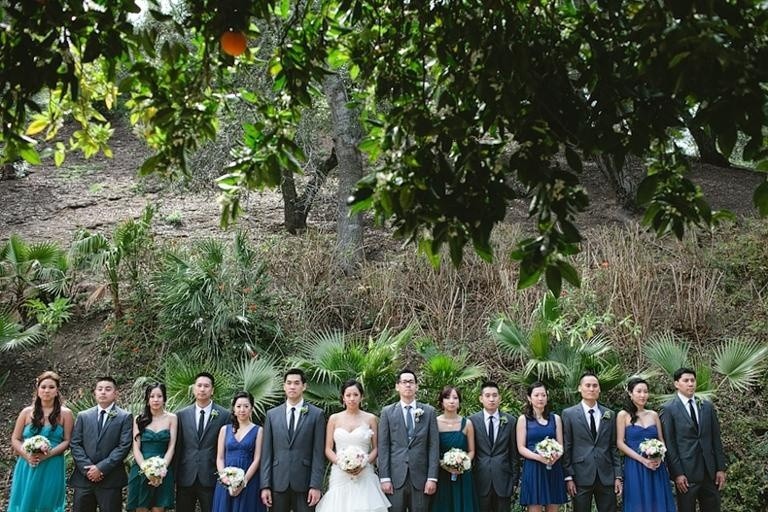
[222,32,247,56]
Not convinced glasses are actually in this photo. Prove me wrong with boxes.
[398,378,416,386]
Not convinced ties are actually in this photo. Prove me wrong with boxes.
[687,399,700,434]
[286,406,297,442]
[586,408,600,443]
[403,405,414,444]
[195,409,205,442]
[485,415,497,448]
[97,409,107,439]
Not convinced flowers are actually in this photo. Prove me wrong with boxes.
[335,446,369,481]
[138,455,168,487]
[215,466,244,495]
[639,438,667,471]
[536,438,563,470]
[22,435,51,468]
[440,448,472,481]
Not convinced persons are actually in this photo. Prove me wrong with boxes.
[562,374,623,512]
[467,381,520,512]
[662,368,726,512]
[516,381,569,512]
[430,386,480,512]
[212,390,266,512]
[69,377,133,512]
[126,382,178,512]
[7,370,73,511]
[615,377,677,512]
[314,379,393,512]
[173,372,232,512]
[378,370,440,512]
[259,368,326,512]
